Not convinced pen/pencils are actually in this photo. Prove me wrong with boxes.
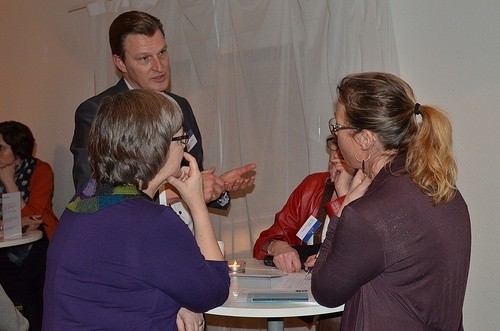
[28,216,50,226]
[305,251,320,276]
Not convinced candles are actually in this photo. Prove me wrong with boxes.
[226,259,246,274]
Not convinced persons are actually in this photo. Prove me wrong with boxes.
[41,88,231,331]
[311,71,472,331]
[252,135,358,331]
[0,120,60,331]
[70,10,256,239]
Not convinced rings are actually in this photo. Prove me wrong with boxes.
[199,322,204,327]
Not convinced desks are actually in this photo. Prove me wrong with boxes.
[205,259,346,331]
[0,220,43,248]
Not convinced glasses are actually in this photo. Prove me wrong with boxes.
[171,132,189,145]
[328,117,361,136]
[326,136,340,150]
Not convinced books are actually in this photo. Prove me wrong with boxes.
[246,291,308,303]
